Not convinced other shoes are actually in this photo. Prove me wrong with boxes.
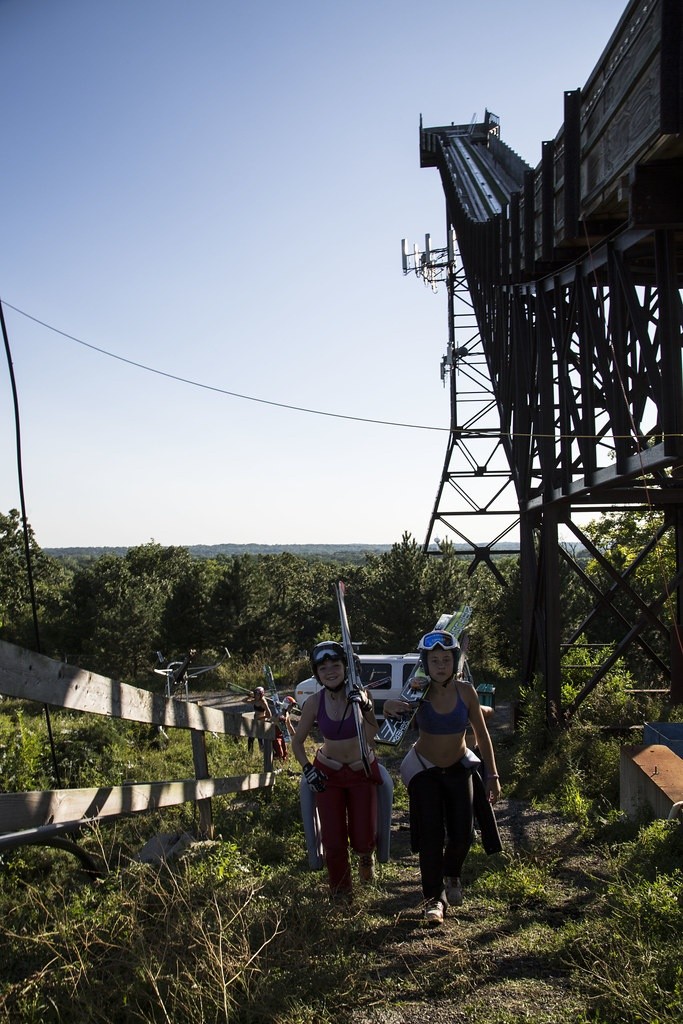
[427,901,444,923]
[445,876,463,904]
[359,853,376,885]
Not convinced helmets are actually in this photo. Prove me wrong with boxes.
[254,687,264,697]
[282,696,298,710]
[420,630,461,676]
[311,641,348,686]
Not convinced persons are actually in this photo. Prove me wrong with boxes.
[241,687,272,755]
[290,641,380,906]
[272,696,297,767]
[383,630,503,923]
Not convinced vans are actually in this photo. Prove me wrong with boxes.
[295,653,474,719]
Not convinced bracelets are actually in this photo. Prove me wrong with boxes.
[486,774,499,779]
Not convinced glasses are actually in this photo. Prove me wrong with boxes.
[418,630,460,651]
[311,643,344,660]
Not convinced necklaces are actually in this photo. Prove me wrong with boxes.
[327,696,341,716]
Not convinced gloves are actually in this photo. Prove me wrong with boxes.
[347,683,373,712]
[303,762,328,793]
